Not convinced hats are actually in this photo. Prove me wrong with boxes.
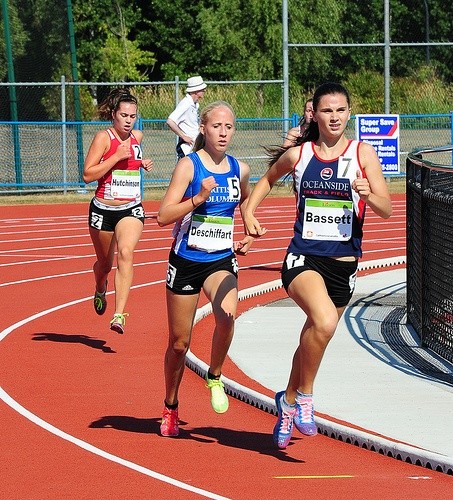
[186,76,207,92]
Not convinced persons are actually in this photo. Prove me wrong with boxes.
[166,76,208,158]
[157,101,254,437]
[283,97,314,149]
[81,88,154,338]
[243,82,392,450]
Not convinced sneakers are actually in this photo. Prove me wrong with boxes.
[93,278,109,315]
[110,313,130,334]
[294,389,318,436]
[205,368,229,413]
[160,405,180,436]
[273,391,297,450]
